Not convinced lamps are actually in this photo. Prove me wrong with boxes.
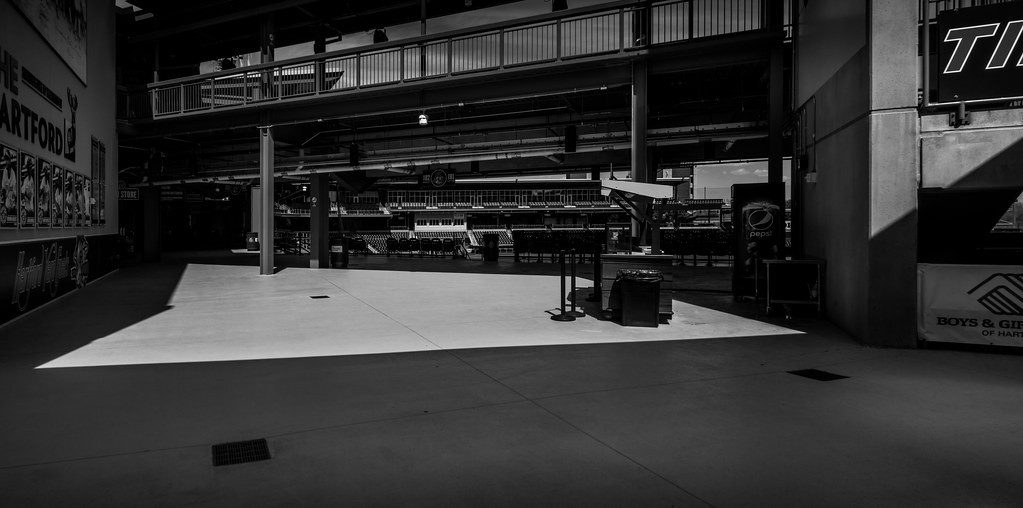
[373,27,389,44]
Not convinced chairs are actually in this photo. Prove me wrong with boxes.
[386,237,455,258]
[672,228,734,266]
[354,237,368,255]
[511,229,604,265]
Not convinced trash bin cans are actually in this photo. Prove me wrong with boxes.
[330,230,350,269]
[482,233,500,261]
[616,268,665,328]
[246,232,260,251]
